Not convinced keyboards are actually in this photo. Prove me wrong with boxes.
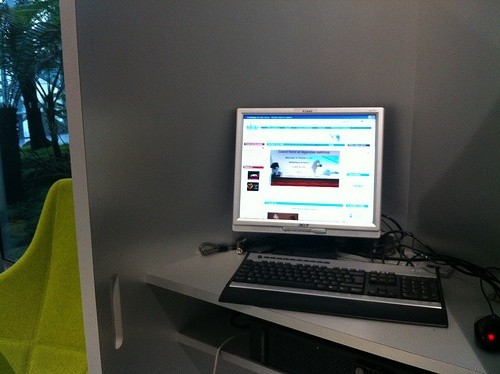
[219,252,449,328]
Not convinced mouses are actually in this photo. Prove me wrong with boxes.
[474,314,500,353]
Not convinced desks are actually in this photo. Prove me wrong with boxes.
[144,243,500,374]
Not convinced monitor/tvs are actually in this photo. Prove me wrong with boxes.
[232,107,385,259]
